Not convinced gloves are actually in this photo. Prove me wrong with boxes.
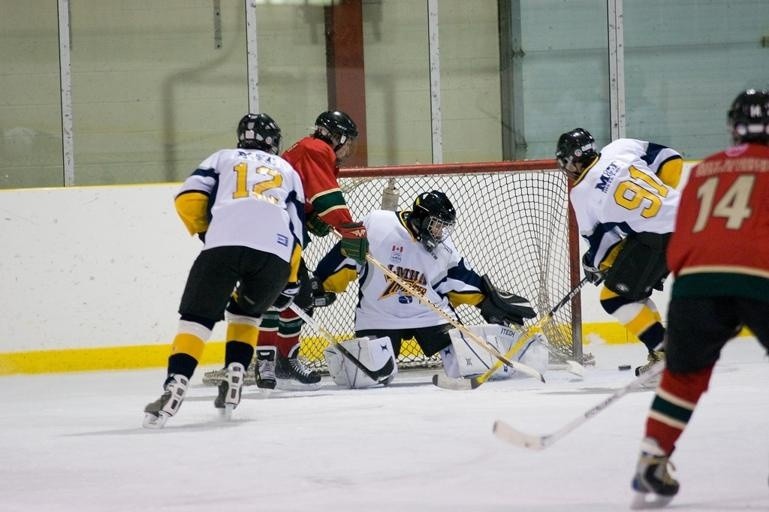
[577,252,606,290]
[472,272,537,332]
[270,278,302,322]
[336,220,372,269]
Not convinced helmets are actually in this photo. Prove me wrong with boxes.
[402,185,460,252]
[234,111,283,159]
[552,127,602,181]
[719,80,769,151]
[306,106,362,169]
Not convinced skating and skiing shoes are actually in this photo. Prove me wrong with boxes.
[140,371,191,433]
[210,360,247,423]
[276,355,321,386]
[252,350,280,392]
[635,351,666,390]
[624,439,689,512]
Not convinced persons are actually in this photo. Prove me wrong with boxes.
[253,111,366,390]
[629,87,769,499]
[308,190,537,387]
[553,126,691,378]
[140,113,306,432]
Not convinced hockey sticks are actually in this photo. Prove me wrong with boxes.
[433,277,592,390]
[492,359,669,450]
[329,225,547,384]
[516,322,588,377]
[289,298,395,382]
